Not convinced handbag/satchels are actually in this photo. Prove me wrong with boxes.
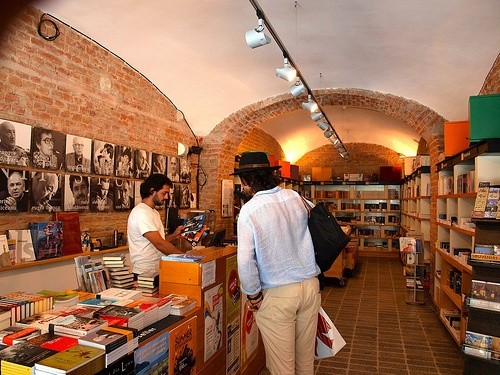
[300,195,351,275]
[314,305,347,360]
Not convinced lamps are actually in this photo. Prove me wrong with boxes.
[329,136,339,144]
[244,18,271,49]
[302,95,318,113]
[288,77,306,98]
[310,111,324,122]
[317,120,329,131]
[275,58,297,82]
[339,151,348,158]
[338,147,345,153]
[323,128,334,138]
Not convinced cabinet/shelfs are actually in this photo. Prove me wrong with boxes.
[0,243,259,375]
[234,139,500,375]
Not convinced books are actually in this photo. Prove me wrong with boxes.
[447,265,462,296]
[440,242,471,260]
[350,228,399,237]
[399,237,421,264]
[0,220,197,375]
[442,171,474,195]
[443,308,462,329]
[324,202,400,210]
[300,189,398,200]
[464,181,500,359]
[334,216,400,223]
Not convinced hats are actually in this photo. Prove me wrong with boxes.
[230,151,283,175]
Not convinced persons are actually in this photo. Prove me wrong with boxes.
[116,180,133,208]
[167,184,191,208]
[0,115,148,177]
[229,152,321,375]
[91,178,114,212]
[30,173,58,211]
[126,173,183,286]
[0,172,28,212]
[65,176,89,209]
[153,155,179,181]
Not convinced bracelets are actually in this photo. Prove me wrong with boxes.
[250,296,263,305]
[247,292,262,300]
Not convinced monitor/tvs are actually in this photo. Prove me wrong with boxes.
[208,227,226,246]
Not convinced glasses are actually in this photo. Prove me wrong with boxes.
[40,138,55,145]
